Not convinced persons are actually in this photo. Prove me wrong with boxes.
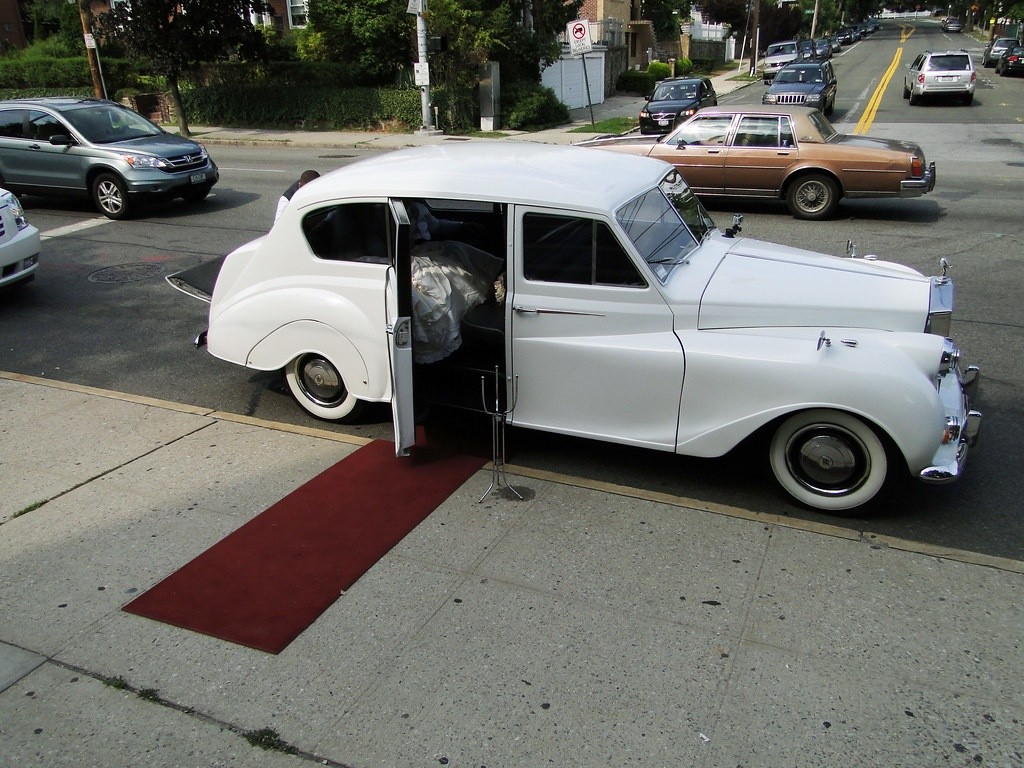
[273,170,320,225]
[693,118,733,146]
[659,87,674,99]
[309,198,503,365]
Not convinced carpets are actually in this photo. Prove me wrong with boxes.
[122,415,523,655]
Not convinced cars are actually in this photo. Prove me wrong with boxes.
[638,77,718,136]
[0,186,42,289]
[941,17,963,33]
[567,104,938,222]
[995,45,1024,78]
[161,136,988,519]
[799,18,881,59]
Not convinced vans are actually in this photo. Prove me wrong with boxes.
[762,40,805,80]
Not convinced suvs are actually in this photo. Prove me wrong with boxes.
[902,49,977,107]
[760,58,839,116]
[981,36,1023,68]
[0,96,220,220]
